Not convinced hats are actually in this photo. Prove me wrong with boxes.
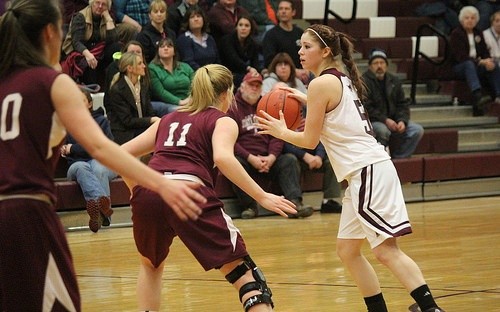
[242,69,264,84]
[367,47,389,66]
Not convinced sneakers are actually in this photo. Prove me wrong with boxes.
[408,302,445,312]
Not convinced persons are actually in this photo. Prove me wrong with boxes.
[253,25,446,312]
[354,48,424,159]
[113,64,298,312]
[0,0,205,312]
[437,0,500,117]
[57,0,341,233]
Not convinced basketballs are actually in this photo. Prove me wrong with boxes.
[255,89,303,134]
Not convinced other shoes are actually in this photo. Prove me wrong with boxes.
[287,203,314,219]
[85,195,114,234]
[474,95,494,116]
[320,199,342,214]
[241,205,258,219]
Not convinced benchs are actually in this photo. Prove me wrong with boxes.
[54,17,500,213]
